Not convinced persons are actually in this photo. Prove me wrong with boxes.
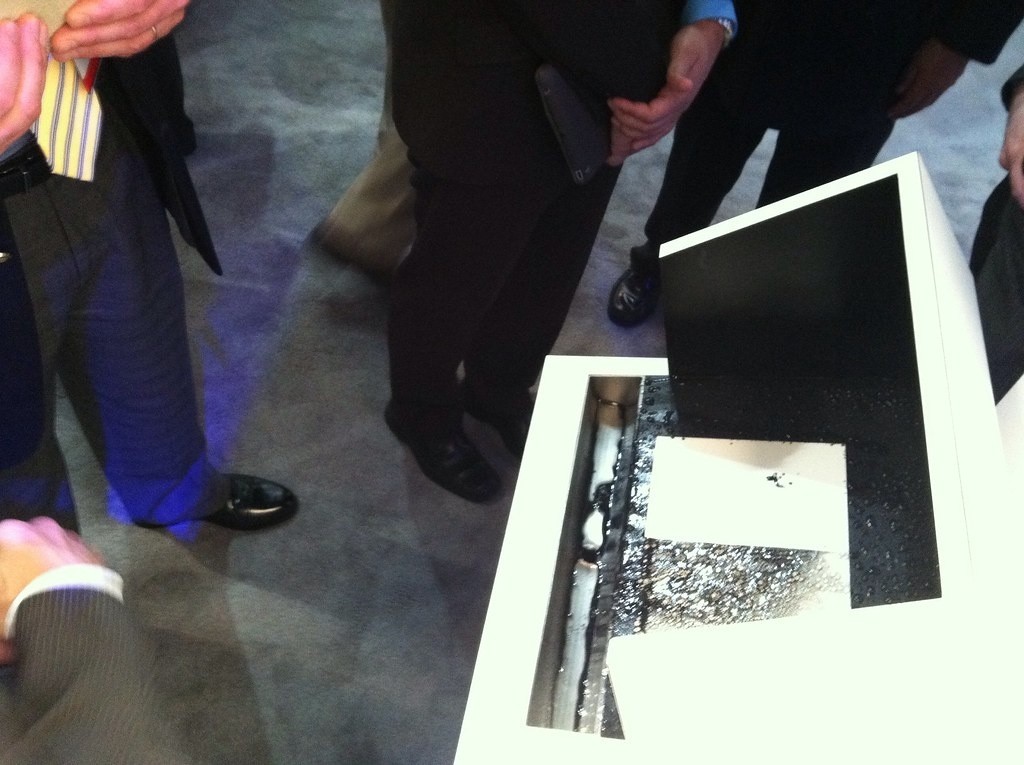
[384,0,738,503]
[968,64,1024,406]
[0,0,297,531]
[608,0,1024,329]
[0,517,188,765]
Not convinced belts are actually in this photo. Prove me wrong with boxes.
[1,150,51,198]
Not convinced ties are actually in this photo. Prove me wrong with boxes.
[27,50,103,182]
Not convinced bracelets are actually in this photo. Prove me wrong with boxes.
[715,17,733,49]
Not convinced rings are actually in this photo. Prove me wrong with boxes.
[152,26,157,41]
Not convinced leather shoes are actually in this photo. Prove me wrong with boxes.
[383,402,502,503]
[465,390,533,458]
[132,473,299,531]
[607,267,657,327]
